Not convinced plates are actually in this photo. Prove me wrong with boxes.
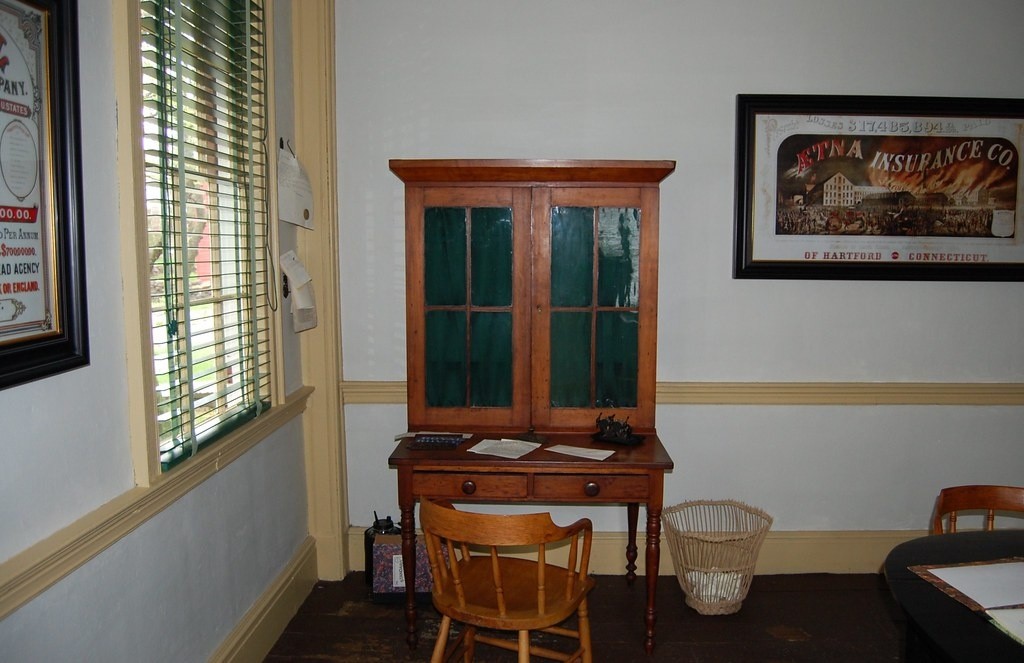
[591,432,646,445]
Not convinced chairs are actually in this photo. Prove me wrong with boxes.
[933,485,1024,535]
[420,494,598,663]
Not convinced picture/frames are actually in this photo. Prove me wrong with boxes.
[732,93,1024,282]
[0,0,91,392]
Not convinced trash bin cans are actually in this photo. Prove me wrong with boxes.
[660,500,773,616]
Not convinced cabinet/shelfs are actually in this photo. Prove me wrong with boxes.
[390,159,677,434]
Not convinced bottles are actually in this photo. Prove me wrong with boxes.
[364,519,402,593]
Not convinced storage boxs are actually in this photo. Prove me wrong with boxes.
[372,533,451,594]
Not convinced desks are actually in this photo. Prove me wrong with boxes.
[388,434,674,656]
[884,529,1024,663]
